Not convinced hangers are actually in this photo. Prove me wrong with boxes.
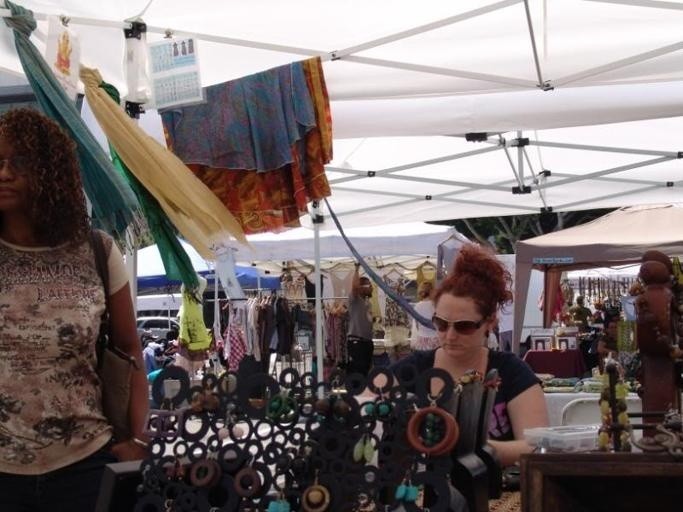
[412,256,439,272]
[324,262,353,272]
[221,301,233,310]
[383,267,407,280]
[244,287,347,306]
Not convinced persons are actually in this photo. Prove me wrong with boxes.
[597,318,619,362]
[0,107,152,512]
[415,280,439,351]
[569,295,594,332]
[374,244,550,467]
[346,263,373,393]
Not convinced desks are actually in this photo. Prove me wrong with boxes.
[518,349,683,511]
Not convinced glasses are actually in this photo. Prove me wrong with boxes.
[432,311,490,335]
[359,285,374,288]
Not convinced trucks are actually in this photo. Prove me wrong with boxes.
[136,292,182,317]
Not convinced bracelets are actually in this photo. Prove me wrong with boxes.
[131,438,149,447]
[406,407,460,456]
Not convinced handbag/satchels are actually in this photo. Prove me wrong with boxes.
[88,231,140,436]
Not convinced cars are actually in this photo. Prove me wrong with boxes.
[135,313,212,345]
